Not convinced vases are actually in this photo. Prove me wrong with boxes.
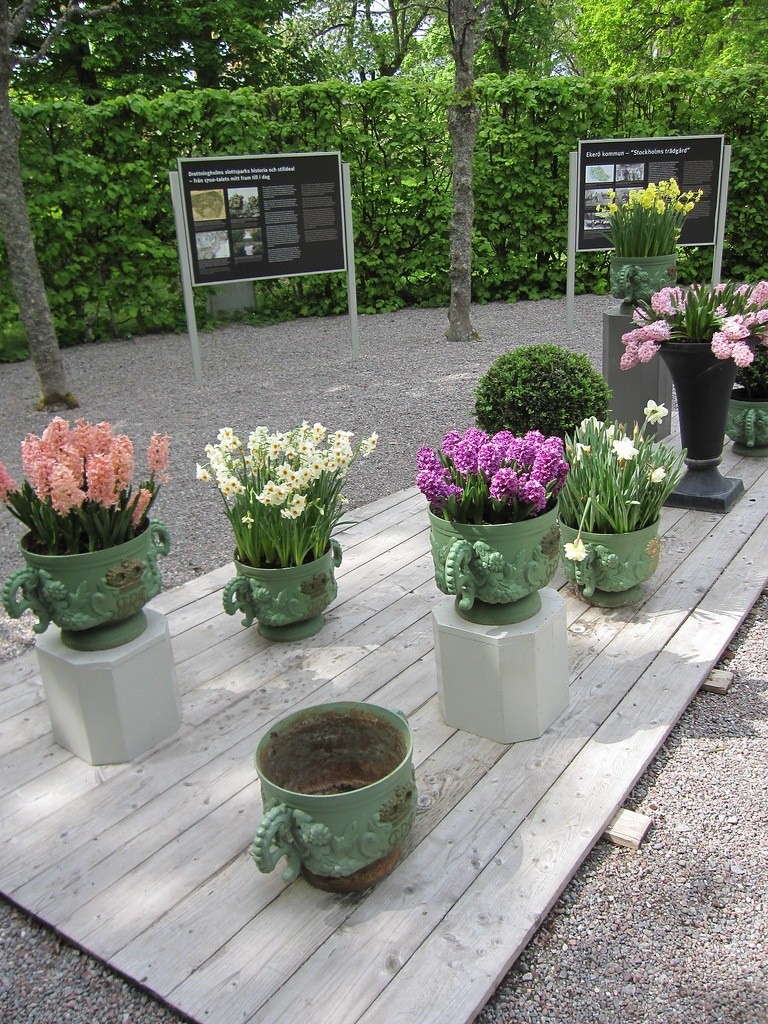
[222,537,343,641]
[252,699,417,893]
[609,252,679,315]
[426,495,561,626]
[652,339,745,515]
[1,516,171,651]
[557,511,661,607]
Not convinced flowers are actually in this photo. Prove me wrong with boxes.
[620,278,768,371]
[416,425,570,523]
[556,400,689,562]
[196,420,379,568]
[597,175,704,257]
[0,414,172,554]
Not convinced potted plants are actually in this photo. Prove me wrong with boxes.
[725,343,768,458]
